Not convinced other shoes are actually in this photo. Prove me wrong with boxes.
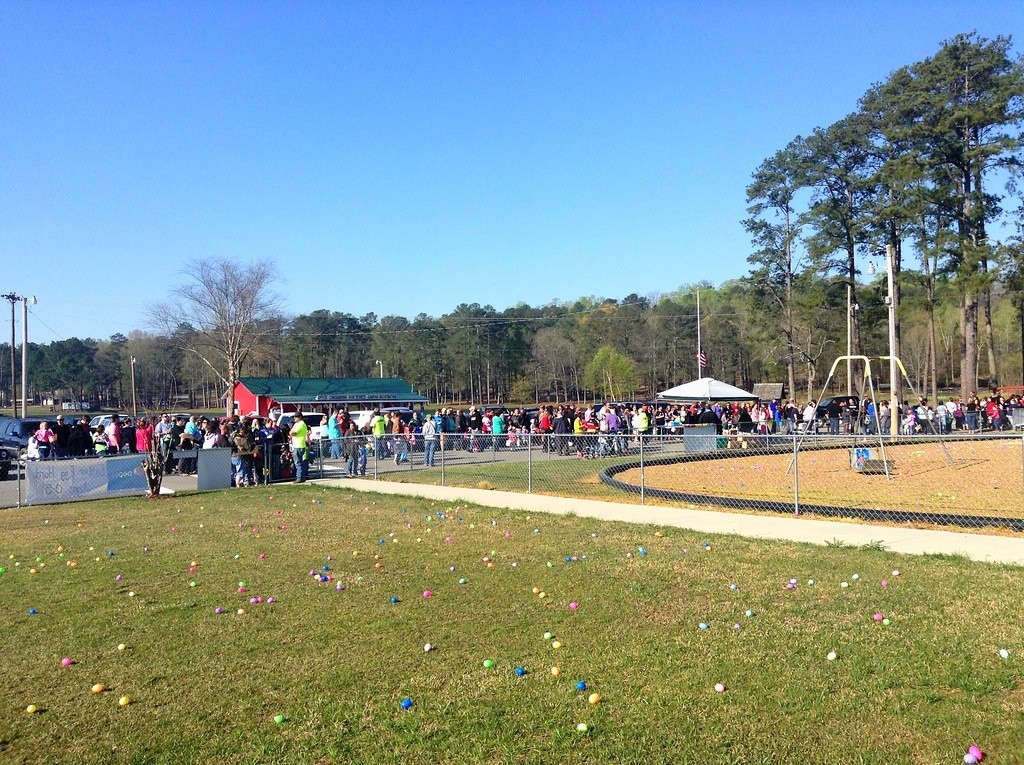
[147,493,159,499]
[358,474,370,477]
[294,480,307,483]
[345,473,360,478]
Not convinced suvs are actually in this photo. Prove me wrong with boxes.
[0,416,82,477]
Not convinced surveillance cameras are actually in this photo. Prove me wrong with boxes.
[855,304,859,311]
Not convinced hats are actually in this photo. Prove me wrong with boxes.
[590,412,598,419]
[673,410,679,416]
[761,402,768,407]
[574,407,586,416]
[424,414,431,420]
[80,415,92,420]
[176,416,183,421]
[56,414,63,420]
[456,408,464,413]
[638,408,645,415]
[441,407,447,413]
[468,405,476,413]
[789,397,795,404]
[111,414,121,422]
[546,405,554,416]
[40,421,48,429]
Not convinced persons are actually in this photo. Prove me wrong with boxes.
[28,391,1024,503]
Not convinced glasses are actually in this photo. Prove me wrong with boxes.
[165,415,169,418]
[203,422,208,424]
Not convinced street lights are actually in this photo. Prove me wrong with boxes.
[866,242,899,442]
[21,295,38,417]
[376,360,383,378]
[847,285,859,396]
[130,355,137,416]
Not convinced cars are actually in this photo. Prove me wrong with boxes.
[347,406,422,438]
[592,401,695,420]
[275,411,328,450]
[89,414,130,429]
[481,405,540,422]
[815,396,863,419]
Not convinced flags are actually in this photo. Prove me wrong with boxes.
[696,345,706,368]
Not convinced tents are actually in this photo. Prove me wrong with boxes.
[657,377,759,410]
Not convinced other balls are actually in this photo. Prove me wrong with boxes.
[0,489,1022,765]
